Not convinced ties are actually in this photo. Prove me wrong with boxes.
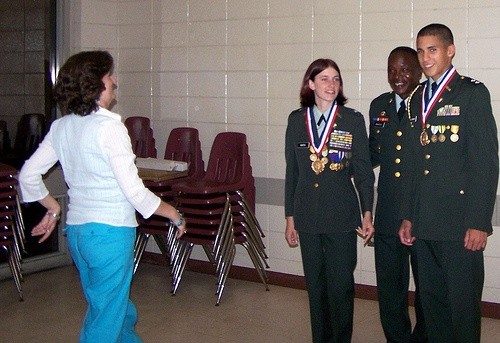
[432,82,437,94]
[398,101,406,121]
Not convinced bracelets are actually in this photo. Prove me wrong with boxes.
[169,210,183,227]
[46,212,60,220]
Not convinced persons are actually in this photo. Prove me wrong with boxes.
[399,23,499,343]
[348,46,422,343]
[283,58,375,343]
[16,51,186,343]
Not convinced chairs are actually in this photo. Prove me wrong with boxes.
[0,118,25,302]
[0,112,48,172]
[124,116,271,307]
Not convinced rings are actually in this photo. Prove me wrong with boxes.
[47,229,49,232]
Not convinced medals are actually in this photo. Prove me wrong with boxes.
[420,132,429,145]
[312,160,324,173]
